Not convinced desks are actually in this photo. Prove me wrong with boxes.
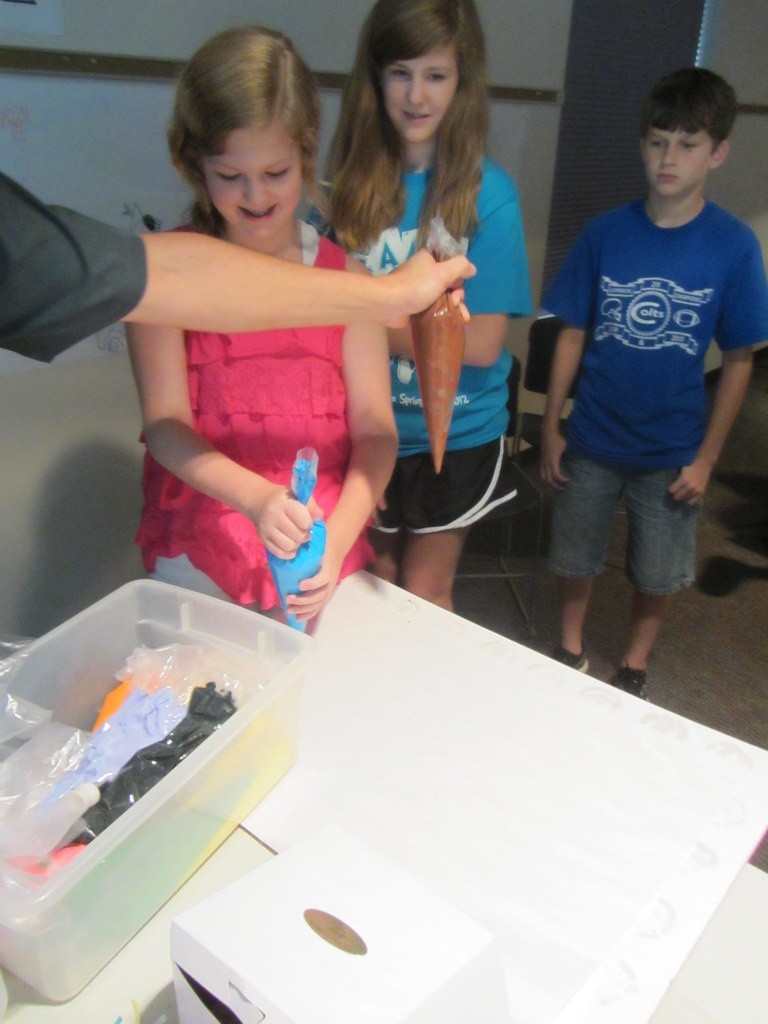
[1,569,768,1024]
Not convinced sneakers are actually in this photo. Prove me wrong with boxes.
[612,664,650,703]
[551,643,589,673]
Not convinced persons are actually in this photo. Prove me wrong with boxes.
[539,68,768,703]
[126,25,400,623]
[0,172,477,365]
[318,0,533,618]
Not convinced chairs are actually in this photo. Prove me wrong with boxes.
[468,310,588,636]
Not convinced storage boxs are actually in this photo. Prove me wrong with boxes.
[1,575,311,1001]
